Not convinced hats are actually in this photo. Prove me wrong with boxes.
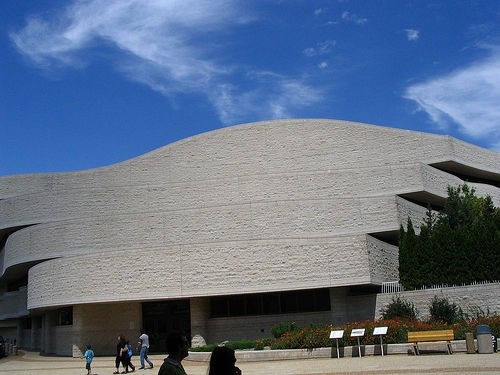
[85,344,91,348]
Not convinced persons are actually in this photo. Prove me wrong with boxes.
[208,346,241,375]
[157,331,190,375]
[81,344,94,375]
[136,329,153,369]
[113,334,135,374]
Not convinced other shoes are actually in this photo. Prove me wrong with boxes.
[139,367,144,370]
[150,364,153,368]
[113,371,119,374]
[133,366,135,371]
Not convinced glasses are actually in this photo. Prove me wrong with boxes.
[234,358,237,362]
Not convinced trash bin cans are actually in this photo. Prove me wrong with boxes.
[477,324,494,354]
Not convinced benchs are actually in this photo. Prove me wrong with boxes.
[405,330,454,356]
